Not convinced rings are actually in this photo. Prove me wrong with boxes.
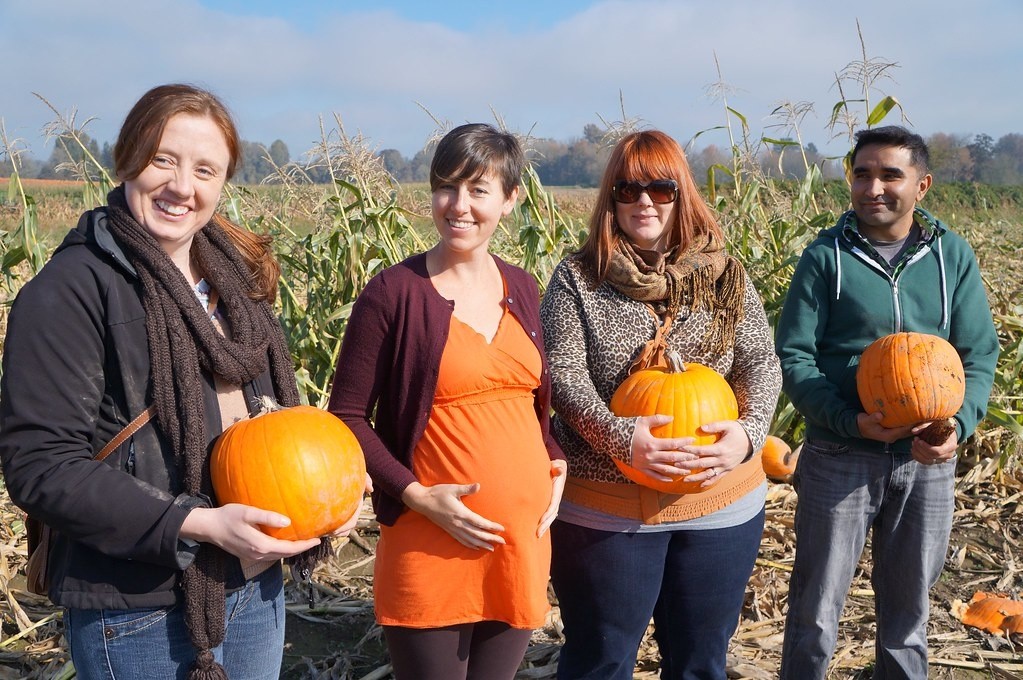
[931,459,937,465]
[712,468,718,476]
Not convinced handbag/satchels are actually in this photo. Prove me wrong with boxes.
[26,514,53,594]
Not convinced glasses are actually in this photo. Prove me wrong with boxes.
[611,180,679,204]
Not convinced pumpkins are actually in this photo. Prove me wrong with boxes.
[857,332,966,429]
[609,351,739,494]
[950,586,1023,635]
[760,435,803,483]
[210,398,367,540]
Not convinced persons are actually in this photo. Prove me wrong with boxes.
[539,131,783,680]
[776,125,998,680]
[328,122,568,680]
[0,84,374,680]
